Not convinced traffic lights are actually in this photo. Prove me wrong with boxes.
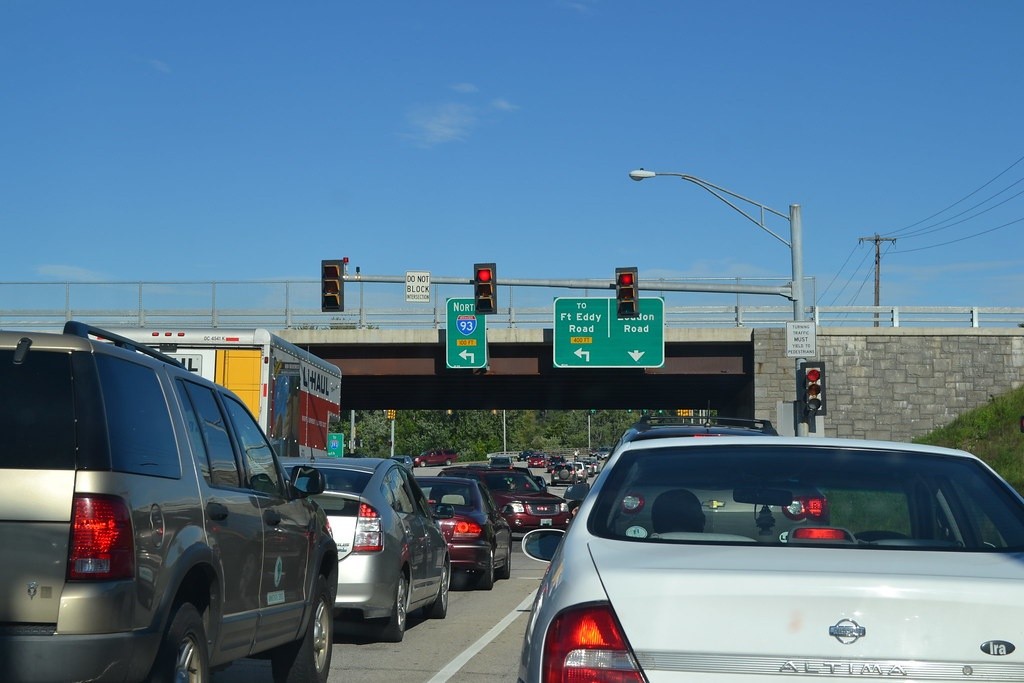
[615,267,639,318]
[321,260,344,312]
[474,263,497,314]
[805,367,823,410]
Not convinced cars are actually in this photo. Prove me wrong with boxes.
[517,450,534,461]
[280,451,452,643]
[528,454,549,468]
[550,464,579,487]
[519,434,1024,683]
[414,475,513,590]
[589,447,599,456]
[583,458,601,474]
[546,455,568,473]
[579,461,594,477]
[436,468,570,538]
[596,446,611,462]
[565,462,589,482]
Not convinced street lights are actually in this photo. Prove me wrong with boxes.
[630,167,811,438]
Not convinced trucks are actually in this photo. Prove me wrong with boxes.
[88,323,343,458]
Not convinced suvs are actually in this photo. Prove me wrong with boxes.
[563,411,830,552]
[413,449,458,467]
[1,318,338,683]
